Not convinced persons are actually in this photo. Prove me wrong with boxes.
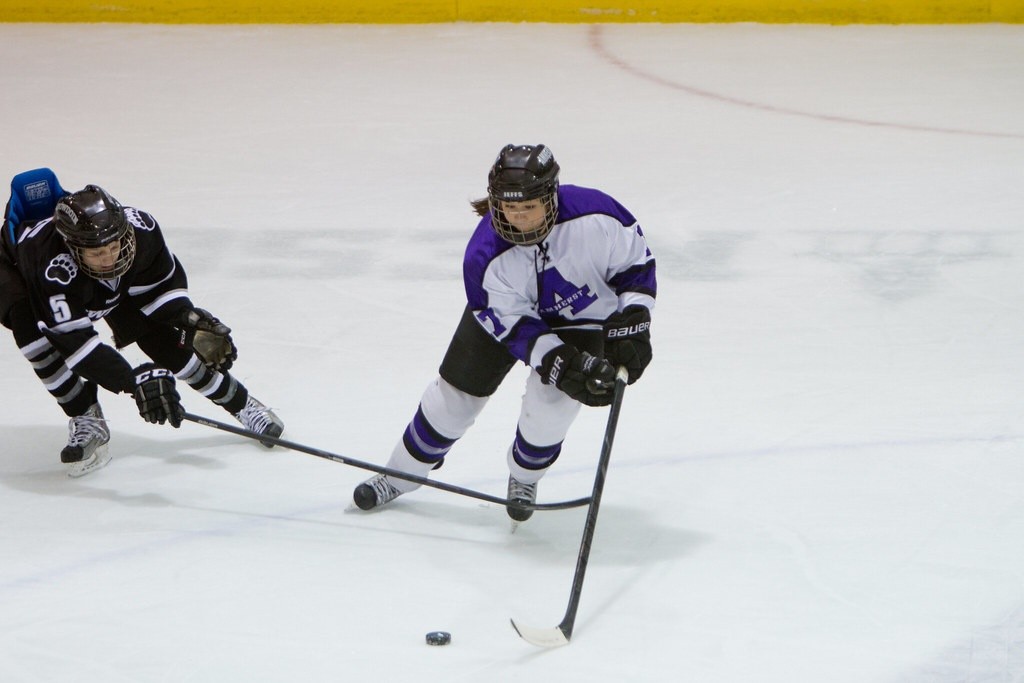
[0,167,289,477]
[345,144,657,532]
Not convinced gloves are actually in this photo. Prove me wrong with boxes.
[131,362,185,428]
[536,344,616,408]
[175,307,239,375]
[603,304,652,387]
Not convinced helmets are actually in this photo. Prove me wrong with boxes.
[54,184,135,279]
[488,144,560,245]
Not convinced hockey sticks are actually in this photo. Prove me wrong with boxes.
[509,363,630,649]
[181,410,591,512]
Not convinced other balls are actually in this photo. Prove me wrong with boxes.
[425,631,452,647]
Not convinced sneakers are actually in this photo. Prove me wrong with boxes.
[236,395,283,447]
[62,402,112,476]
[507,474,537,534]
[352,473,403,511]
